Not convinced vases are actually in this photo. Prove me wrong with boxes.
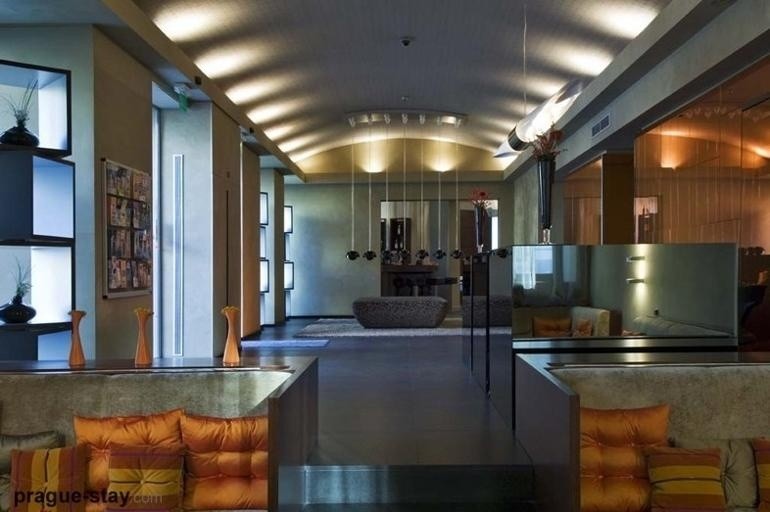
[67,310,87,366]
[222,308,240,363]
[134,307,153,365]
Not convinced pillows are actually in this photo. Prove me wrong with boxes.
[573,318,593,337]
[1,409,271,511]
[579,405,770,512]
[534,316,572,337]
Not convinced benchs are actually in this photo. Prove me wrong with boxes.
[352,296,448,327]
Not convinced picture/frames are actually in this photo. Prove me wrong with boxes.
[99,156,153,300]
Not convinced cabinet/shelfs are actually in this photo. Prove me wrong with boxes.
[390,218,411,252]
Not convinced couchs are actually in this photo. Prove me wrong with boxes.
[489,315,736,428]
[512,306,622,338]
[0,357,317,511]
[515,353,770,512]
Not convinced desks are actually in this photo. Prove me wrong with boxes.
[381,264,438,296]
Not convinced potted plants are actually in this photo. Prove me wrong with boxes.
[529,123,568,245]
[0,256,36,324]
[469,191,491,254]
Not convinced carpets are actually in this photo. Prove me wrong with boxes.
[293,317,513,337]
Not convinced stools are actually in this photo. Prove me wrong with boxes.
[462,295,512,327]
